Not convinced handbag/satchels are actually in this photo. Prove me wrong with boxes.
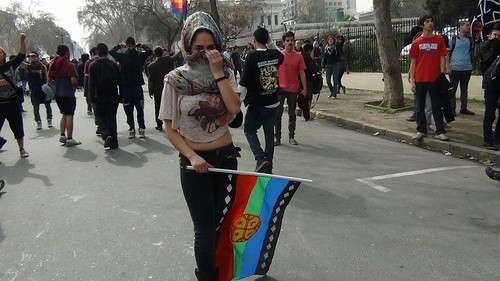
[15,84,24,103]
[312,74,323,94]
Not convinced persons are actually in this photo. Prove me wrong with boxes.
[158,11,243,281]
[47,45,82,147]
[0,33,54,191]
[222,43,256,83]
[276,29,346,121]
[71,43,125,149]
[406,16,449,141]
[273,31,307,147]
[479,23,500,181]
[238,26,284,174]
[108,36,153,139]
[446,21,475,117]
[143,47,187,130]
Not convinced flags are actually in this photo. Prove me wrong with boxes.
[214,172,301,281]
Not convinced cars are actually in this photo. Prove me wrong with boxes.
[401,27,462,62]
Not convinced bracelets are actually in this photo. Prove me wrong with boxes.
[215,76,227,82]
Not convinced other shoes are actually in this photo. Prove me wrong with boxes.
[128,129,135,139]
[66,139,81,146]
[483,142,497,149]
[343,86,345,94]
[289,137,298,145]
[138,128,145,138]
[60,136,66,142]
[88,111,92,115]
[274,139,281,146]
[328,93,336,99]
[37,122,42,130]
[460,108,475,115]
[22,109,27,112]
[255,158,270,173]
[0,179,5,191]
[20,149,28,158]
[47,119,53,127]
[96,126,101,135]
[104,136,112,148]
[485,167,500,181]
[405,116,451,140]
[155,127,162,130]
